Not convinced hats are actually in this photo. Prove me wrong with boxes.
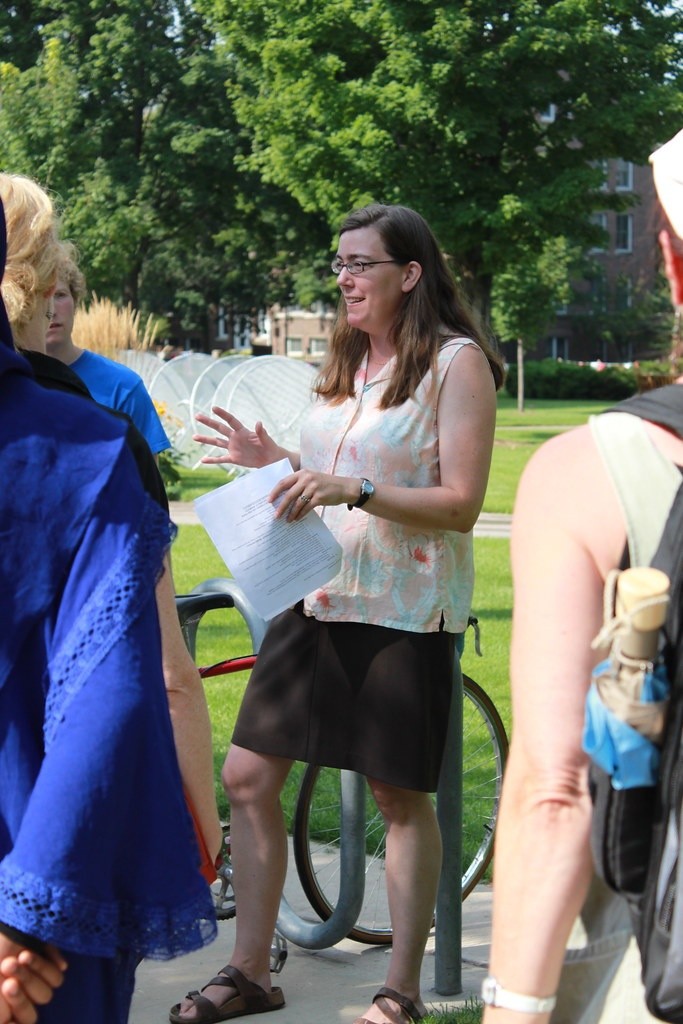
[647,124,683,245]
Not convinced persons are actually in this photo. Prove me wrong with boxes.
[482,129,683,1024]
[0,175,223,1024]
[170,205,506,1024]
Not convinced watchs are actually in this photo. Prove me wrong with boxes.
[348,478,374,511]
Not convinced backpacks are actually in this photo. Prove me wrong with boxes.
[594,382,683,1024]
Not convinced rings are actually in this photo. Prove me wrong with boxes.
[300,495,310,503]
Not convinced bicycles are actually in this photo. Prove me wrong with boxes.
[173,590,512,973]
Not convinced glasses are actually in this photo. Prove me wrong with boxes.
[330,257,403,276]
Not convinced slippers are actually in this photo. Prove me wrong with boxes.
[169,965,285,1024]
[352,987,422,1024]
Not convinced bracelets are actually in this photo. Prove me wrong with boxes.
[481,977,558,1013]
